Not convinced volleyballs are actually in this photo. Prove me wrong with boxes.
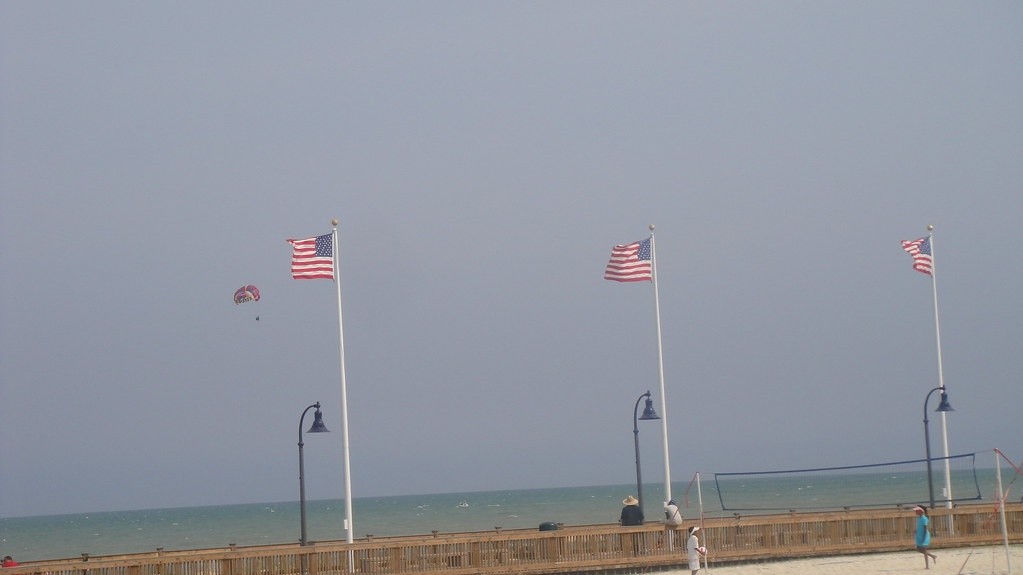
[698,547,708,556]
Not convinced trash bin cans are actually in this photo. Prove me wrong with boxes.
[538,521,559,561]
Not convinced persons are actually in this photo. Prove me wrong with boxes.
[659,500,682,526]
[2,556,23,575]
[686,527,705,575]
[621,496,644,526]
[913,505,937,569]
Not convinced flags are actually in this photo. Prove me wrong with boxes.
[604,238,652,282]
[902,237,932,274]
[287,233,334,279]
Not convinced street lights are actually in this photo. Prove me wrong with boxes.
[923,384,957,538]
[297,403,332,574]
[633,393,662,555]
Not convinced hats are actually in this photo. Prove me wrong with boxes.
[689,527,700,535]
[623,496,638,505]
[669,500,676,505]
[912,506,925,513]
